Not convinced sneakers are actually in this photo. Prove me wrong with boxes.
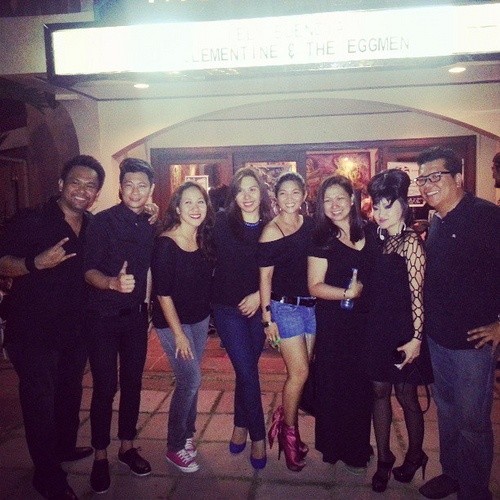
[117,447,153,474]
[90,458,110,494]
[184,438,197,457]
[165,447,198,472]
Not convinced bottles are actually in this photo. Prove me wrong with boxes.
[341,267,358,309]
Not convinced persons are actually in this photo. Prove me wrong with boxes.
[0,153,163,499]
[491,153,499,189]
[257,172,319,472]
[306,175,387,469]
[150,181,214,475]
[206,169,274,470]
[82,156,160,497]
[413,146,499,500]
[359,169,434,494]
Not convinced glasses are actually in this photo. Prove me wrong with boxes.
[415,171,450,186]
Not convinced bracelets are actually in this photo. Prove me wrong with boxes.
[263,319,275,327]
[343,287,347,300]
[258,305,271,313]
[25,255,42,273]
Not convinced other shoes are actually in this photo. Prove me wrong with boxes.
[323,453,342,466]
[347,460,367,473]
[49,483,78,500]
[64,446,92,461]
[420,474,458,499]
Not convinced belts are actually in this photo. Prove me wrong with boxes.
[271,293,316,307]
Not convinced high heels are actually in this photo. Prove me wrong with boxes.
[395,451,429,484]
[230,426,248,454]
[272,404,308,456]
[268,421,307,472]
[373,450,396,492]
[250,440,267,469]
